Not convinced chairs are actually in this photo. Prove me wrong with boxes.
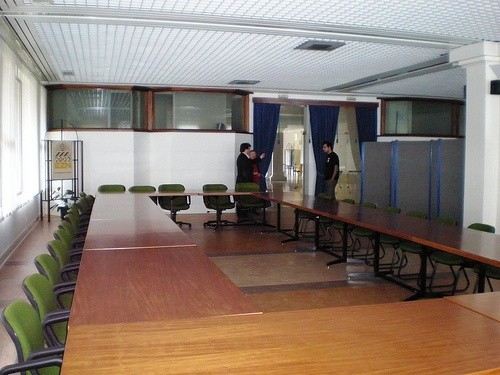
[98,182,272,228]
[293,193,500,294]
[0,190,95,375]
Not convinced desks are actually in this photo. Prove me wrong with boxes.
[61,189,500,375]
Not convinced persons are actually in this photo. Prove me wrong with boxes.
[322,141,340,200]
[237,142,266,217]
[248,150,261,184]
